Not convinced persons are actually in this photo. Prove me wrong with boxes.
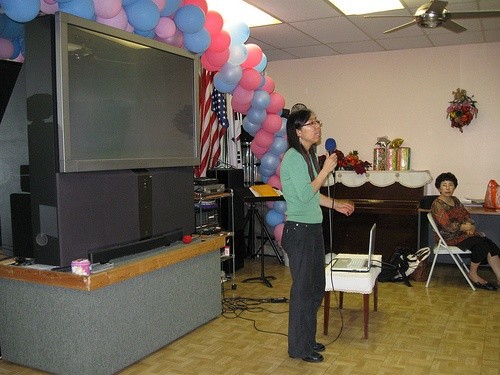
[279,110,354,363]
[430,173,500,291]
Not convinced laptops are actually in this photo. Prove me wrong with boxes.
[332,223,377,272]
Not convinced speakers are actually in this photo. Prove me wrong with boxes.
[208,168,244,273]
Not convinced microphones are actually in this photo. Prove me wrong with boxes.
[325,138,336,177]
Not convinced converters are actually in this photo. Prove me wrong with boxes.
[271,297,287,303]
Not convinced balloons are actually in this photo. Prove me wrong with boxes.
[265,201,287,248]
[173,0,267,93]
[231,76,288,190]
[0,0,183,63]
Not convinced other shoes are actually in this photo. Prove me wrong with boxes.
[289,352,324,362]
[476,282,497,291]
[313,343,325,351]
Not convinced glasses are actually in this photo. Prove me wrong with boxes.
[301,120,322,128]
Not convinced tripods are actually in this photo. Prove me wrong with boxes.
[241,143,284,288]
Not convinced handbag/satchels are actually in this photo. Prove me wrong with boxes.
[484,180,500,209]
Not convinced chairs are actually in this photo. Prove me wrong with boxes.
[425,213,477,291]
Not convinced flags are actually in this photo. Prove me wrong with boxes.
[195,68,229,176]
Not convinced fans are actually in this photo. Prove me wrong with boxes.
[364,0,500,34]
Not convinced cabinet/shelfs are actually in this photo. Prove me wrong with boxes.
[320,170,432,261]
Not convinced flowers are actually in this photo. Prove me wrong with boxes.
[445,88,479,133]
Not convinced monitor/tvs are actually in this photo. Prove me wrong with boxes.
[54,10,201,173]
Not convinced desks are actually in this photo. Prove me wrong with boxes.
[417,205,500,266]
[323,254,382,339]
